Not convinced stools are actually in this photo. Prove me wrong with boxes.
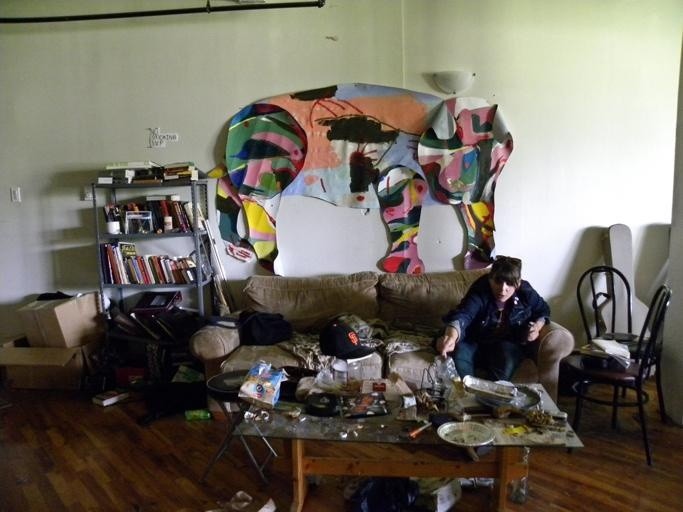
[198,369,278,485]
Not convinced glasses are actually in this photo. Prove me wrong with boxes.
[494,255,522,269]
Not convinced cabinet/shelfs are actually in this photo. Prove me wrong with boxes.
[90,177,215,317]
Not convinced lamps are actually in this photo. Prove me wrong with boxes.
[432,71,476,94]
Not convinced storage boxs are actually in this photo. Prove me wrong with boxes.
[0,290,104,390]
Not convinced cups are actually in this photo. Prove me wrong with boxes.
[107,221,120,235]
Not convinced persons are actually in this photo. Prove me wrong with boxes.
[430,255,551,465]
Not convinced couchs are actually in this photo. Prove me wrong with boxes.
[188,268,574,413]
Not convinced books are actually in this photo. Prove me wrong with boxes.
[87,158,207,408]
[580,338,631,369]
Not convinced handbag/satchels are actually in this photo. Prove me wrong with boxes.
[238,307,293,346]
[319,312,386,359]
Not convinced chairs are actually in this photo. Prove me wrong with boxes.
[566,264,673,466]
